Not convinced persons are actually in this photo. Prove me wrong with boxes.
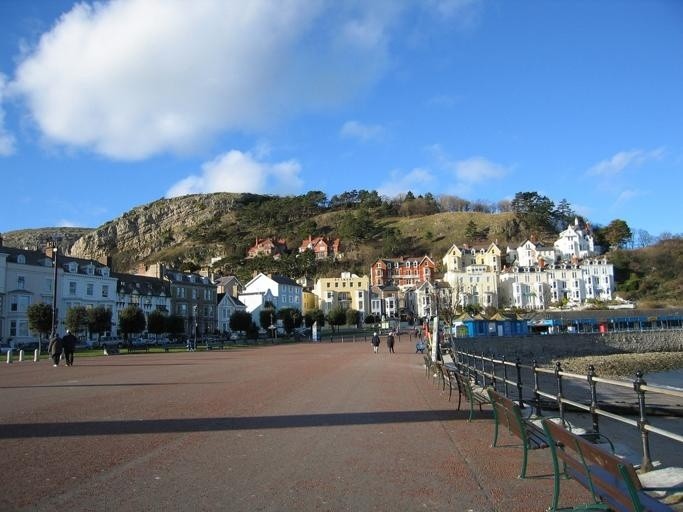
[46,333,62,367]
[61,328,77,366]
[369,331,379,354]
[385,331,395,355]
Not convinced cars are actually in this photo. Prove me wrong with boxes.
[230,326,321,341]
[0,331,227,352]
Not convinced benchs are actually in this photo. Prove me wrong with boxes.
[128,342,149,353]
[424,355,673,512]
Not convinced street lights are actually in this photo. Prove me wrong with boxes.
[192,302,199,350]
[369,282,398,328]
[50,247,59,352]
[433,280,443,381]
